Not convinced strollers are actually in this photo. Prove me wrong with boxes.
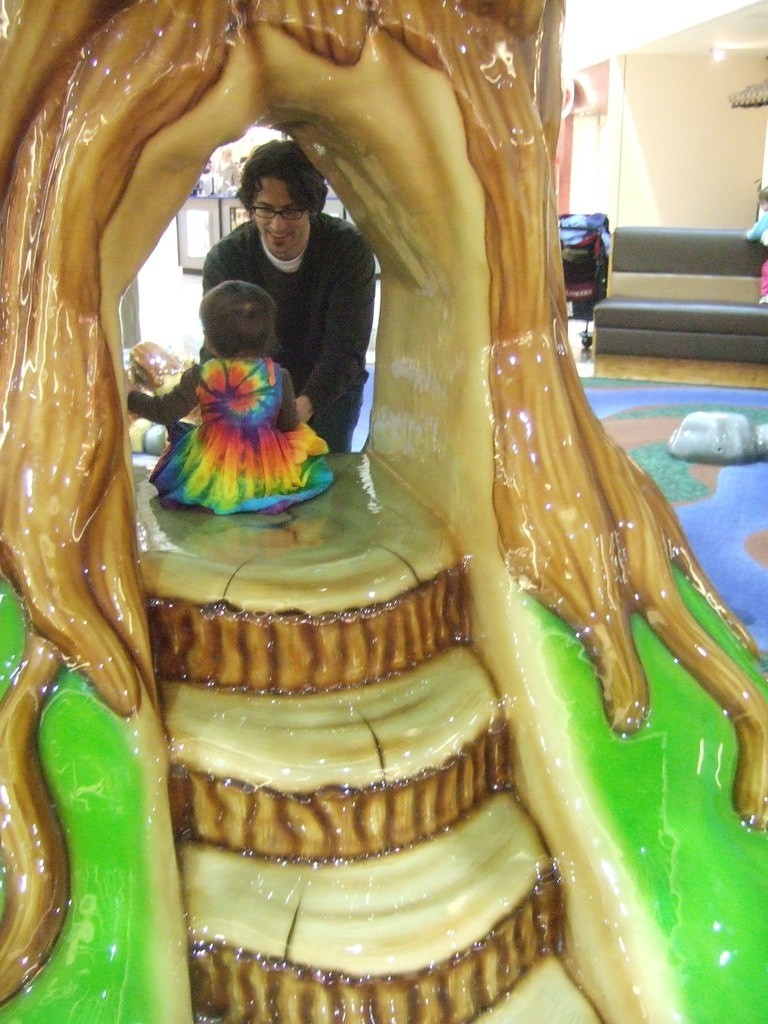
[558,212,611,349]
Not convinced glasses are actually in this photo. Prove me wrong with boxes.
[251,205,307,220]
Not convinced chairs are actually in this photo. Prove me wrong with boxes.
[556,213,611,348]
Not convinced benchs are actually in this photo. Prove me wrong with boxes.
[592,225,767,362]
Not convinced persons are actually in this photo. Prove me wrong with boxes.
[744,187,768,304]
[125,280,335,515]
[197,139,375,452]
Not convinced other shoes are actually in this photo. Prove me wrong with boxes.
[758,297,767,304]
